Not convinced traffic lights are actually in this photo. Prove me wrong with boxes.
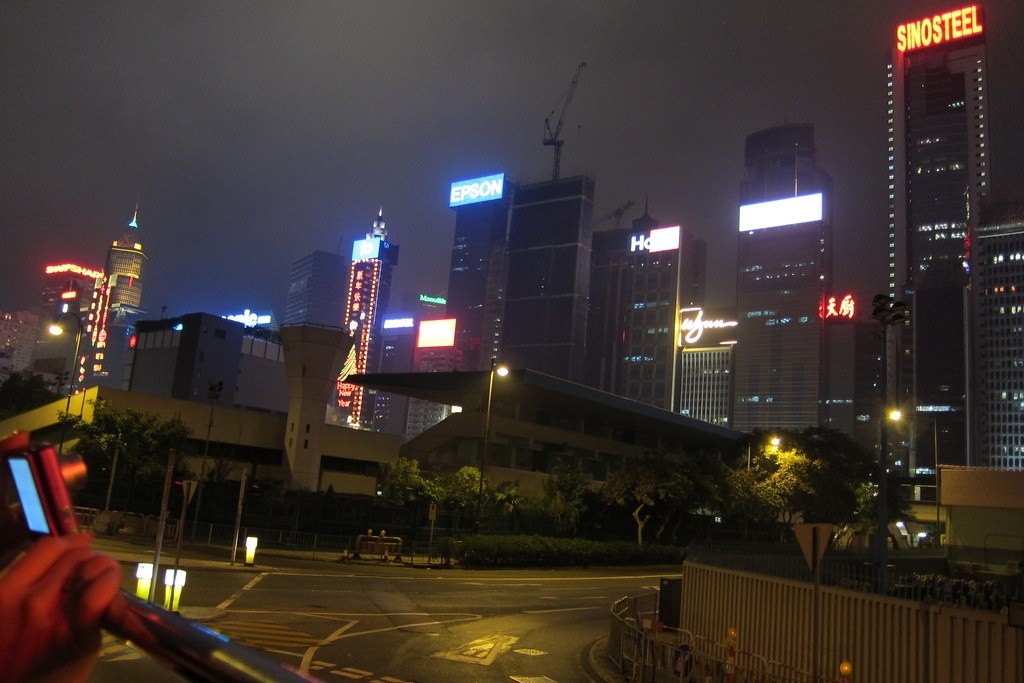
[168,469,184,504]
[374,473,386,499]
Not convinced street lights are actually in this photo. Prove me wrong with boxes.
[470,358,509,530]
[49,311,84,452]
[871,294,906,594]
[246,475,262,505]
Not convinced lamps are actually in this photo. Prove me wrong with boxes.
[245,537,258,563]
[137,563,153,600]
[164,569,186,611]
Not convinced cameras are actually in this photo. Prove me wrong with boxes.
[5,443,78,545]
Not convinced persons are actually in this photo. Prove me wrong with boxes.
[0,429,122,683]
